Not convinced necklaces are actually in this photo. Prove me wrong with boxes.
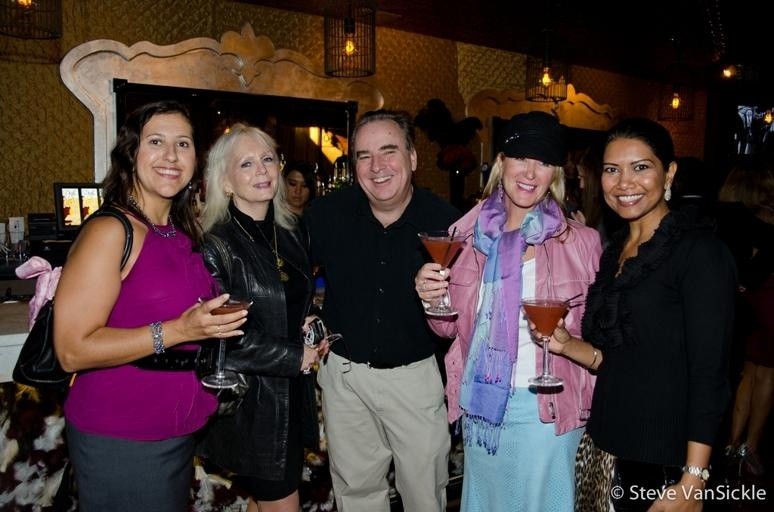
[128,197,176,238]
[253,221,289,282]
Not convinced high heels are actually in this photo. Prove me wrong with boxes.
[721,445,735,471]
[737,445,766,478]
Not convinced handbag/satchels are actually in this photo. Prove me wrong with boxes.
[12,209,134,391]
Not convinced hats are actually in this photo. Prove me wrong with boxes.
[499,111,571,166]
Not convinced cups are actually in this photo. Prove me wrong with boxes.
[417,229,466,317]
[520,296,570,388]
[197,295,255,391]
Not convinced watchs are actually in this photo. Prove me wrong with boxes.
[681,465,712,481]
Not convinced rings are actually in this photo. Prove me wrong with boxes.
[419,282,427,293]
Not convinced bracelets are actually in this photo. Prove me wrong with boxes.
[148,321,167,356]
[589,347,597,369]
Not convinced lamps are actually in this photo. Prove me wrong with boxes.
[526,28,568,102]
[657,82,693,122]
[324,0,376,78]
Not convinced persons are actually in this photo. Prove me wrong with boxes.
[416,109,605,512]
[200,125,329,512]
[281,157,319,221]
[522,117,739,512]
[720,164,774,476]
[302,111,463,512]
[56,102,247,512]
